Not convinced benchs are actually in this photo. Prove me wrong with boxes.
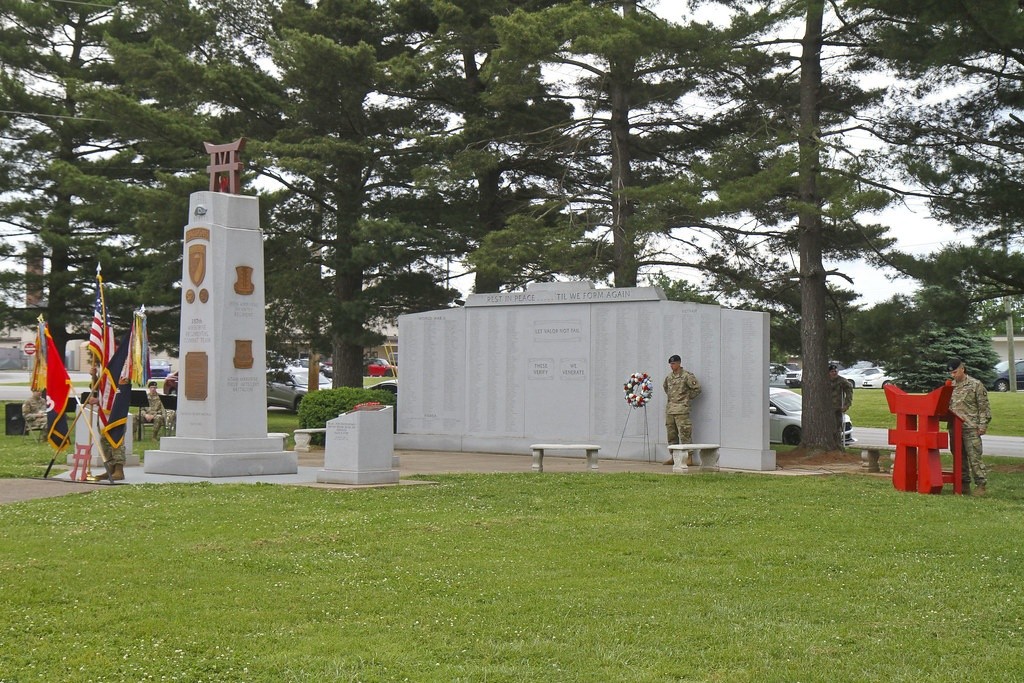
[668,443,721,474]
[849,426,1024,476]
[528,443,601,473]
[293,427,326,452]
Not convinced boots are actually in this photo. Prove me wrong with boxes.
[96,465,115,479]
[970,482,986,497]
[687,454,693,466]
[663,459,674,465]
[961,482,971,493]
[151,433,158,442]
[108,464,125,480]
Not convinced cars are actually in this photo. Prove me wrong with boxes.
[770,386,854,447]
[266,352,398,411]
[150,357,173,378]
[986,358,1024,393]
[770,358,902,389]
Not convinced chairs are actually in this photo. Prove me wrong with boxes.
[138,406,167,440]
[23,419,47,445]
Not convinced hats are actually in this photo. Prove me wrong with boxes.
[829,364,838,370]
[945,359,961,372]
[148,382,157,388]
[669,355,681,363]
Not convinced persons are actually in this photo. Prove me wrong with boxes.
[947,359,992,497]
[22,388,48,442]
[829,365,853,412]
[89,368,126,480]
[663,355,702,466]
[133,382,163,441]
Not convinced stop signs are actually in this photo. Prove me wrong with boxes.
[24,341,37,356]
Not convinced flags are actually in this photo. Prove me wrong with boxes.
[31,322,72,451]
[88,276,152,449]
